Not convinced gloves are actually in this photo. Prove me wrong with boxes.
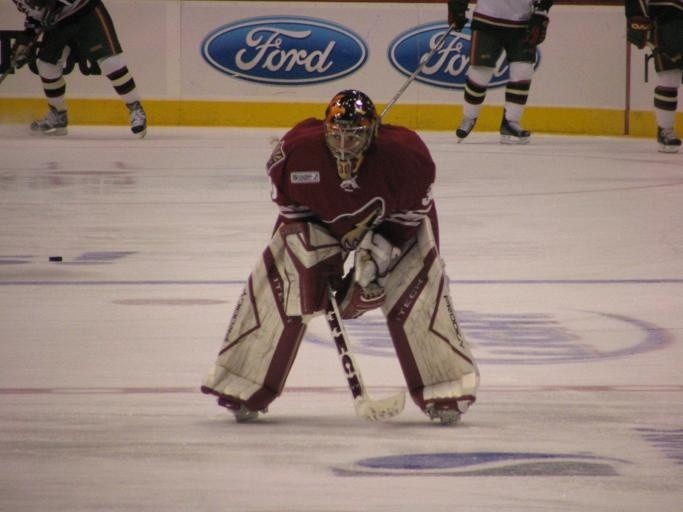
[627,15,654,50]
[523,10,550,47]
[447,6,471,33]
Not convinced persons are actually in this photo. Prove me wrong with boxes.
[10,0,148,139]
[446,1,553,145]
[625,1,682,153]
[195,89,481,427]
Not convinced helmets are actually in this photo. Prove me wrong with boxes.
[324,89,380,131]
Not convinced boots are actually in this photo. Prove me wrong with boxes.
[29,111,69,131]
[499,119,532,137]
[126,100,148,134]
[656,124,682,146]
[456,116,477,138]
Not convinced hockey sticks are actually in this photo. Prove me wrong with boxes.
[320,283,406,425]
